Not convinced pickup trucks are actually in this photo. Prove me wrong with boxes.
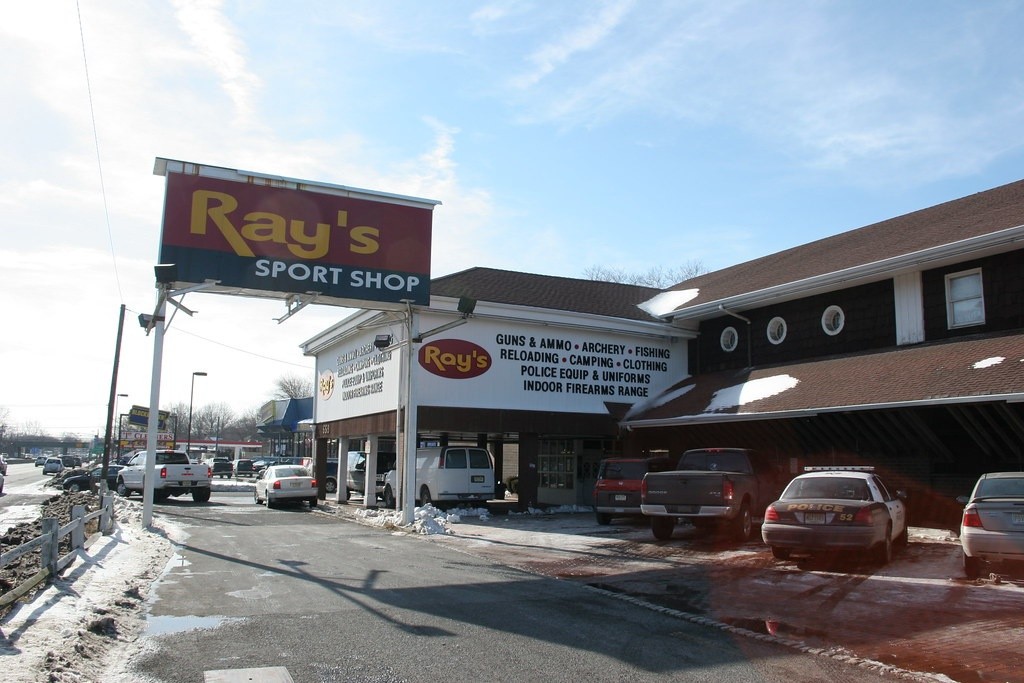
[116,450,212,504]
[640,448,781,543]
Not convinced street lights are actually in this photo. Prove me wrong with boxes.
[188,373,207,457]
[112,394,128,463]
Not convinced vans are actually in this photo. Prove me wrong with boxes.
[383,446,496,509]
[347,451,396,503]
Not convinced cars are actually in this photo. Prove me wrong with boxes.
[761,466,908,567]
[199,458,233,479]
[62,465,124,493]
[232,456,338,493]
[254,464,318,509]
[25,453,83,475]
[957,472,1024,577]
[593,456,679,526]
[0,453,9,493]
